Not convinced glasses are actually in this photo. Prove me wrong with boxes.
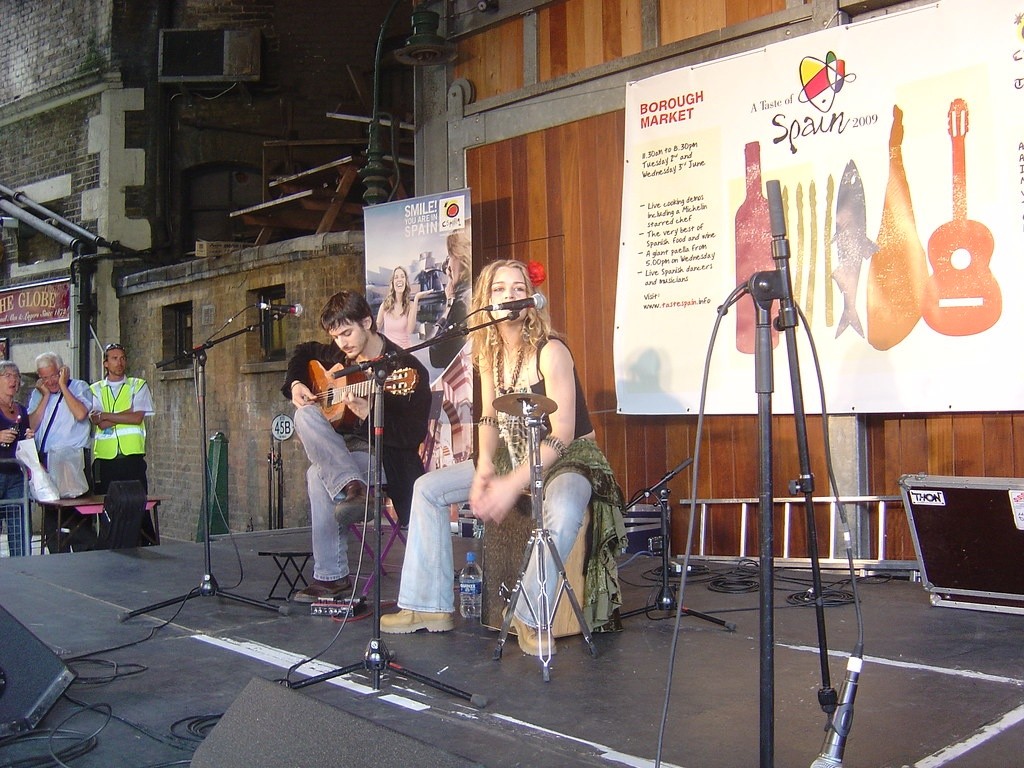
[0,372,20,379]
[105,343,124,361]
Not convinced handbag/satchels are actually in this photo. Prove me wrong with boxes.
[14,439,89,503]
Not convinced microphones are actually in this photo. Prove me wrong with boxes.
[254,303,304,317]
[809,642,865,768]
[483,293,547,311]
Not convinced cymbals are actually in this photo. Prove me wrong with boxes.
[492,392,558,418]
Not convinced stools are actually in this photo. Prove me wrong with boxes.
[259,551,313,603]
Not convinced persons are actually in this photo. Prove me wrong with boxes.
[429,217,472,369]
[281,290,433,603]
[88,343,155,500]
[379,260,598,657]
[375,266,434,350]
[27,353,97,553]
[0,360,35,557]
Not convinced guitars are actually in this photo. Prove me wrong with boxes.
[306,359,420,434]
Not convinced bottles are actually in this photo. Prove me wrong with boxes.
[0,415,21,448]
[459,551,482,618]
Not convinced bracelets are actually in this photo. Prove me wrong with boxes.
[479,416,499,428]
[290,381,301,390]
[542,434,566,458]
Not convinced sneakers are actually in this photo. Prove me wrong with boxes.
[501,604,556,656]
[294,575,353,602]
[334,480,375,523]
[379,609,456,634]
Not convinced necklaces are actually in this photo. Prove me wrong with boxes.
[496,342,524,394]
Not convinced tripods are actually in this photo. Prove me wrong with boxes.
[493,402,597,682]
[281,302,522,707]
[118,305,292,622]
[609,459,737,630]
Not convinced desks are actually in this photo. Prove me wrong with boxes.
[39,494,175,555]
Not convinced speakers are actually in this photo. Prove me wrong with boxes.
[0,607,79,737]
[191,676,484,768]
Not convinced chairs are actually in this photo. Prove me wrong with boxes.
[342,412,438,595]
